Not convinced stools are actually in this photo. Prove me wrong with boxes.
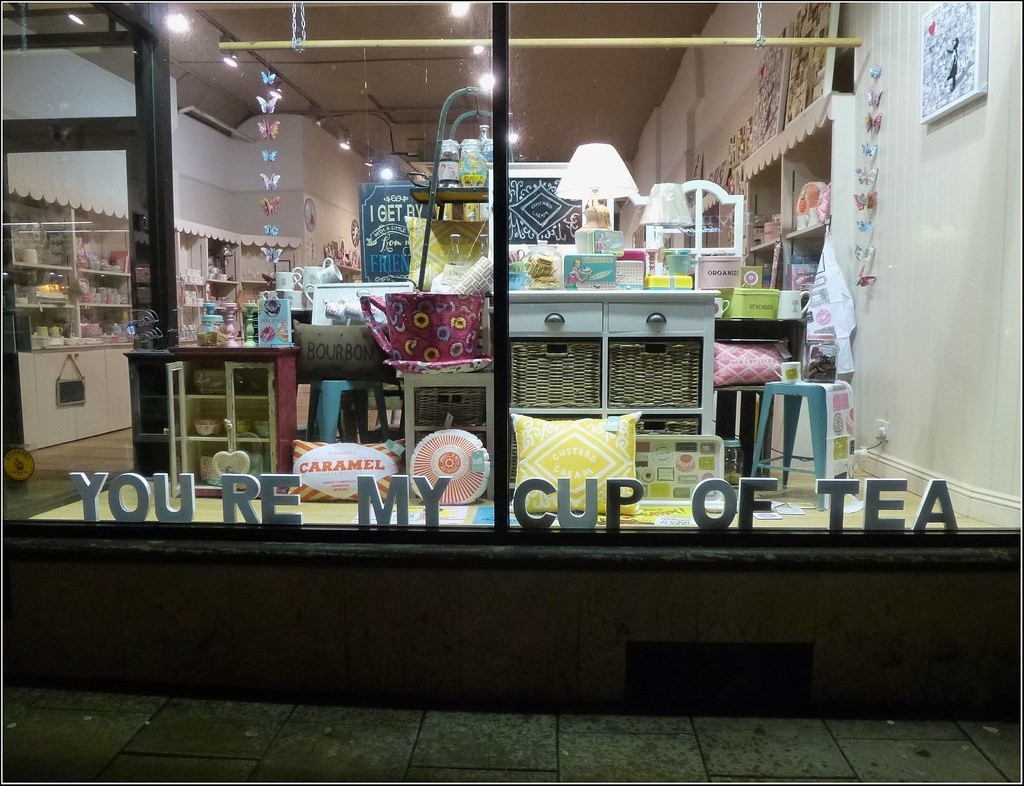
[751,382,853,512]
[305,379,389,444]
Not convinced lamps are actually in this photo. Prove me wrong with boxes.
[554,143,639,229]
[639,182,694,227]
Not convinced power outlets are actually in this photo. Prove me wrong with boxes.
[875,419,889,441]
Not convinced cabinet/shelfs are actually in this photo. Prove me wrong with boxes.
[403,372,494,503]
[493,290,720,490]
[171,340,299,497]
[8,175,132,452]
[740,94,855,296]
[174,219,301,346]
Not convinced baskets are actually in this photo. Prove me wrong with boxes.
[606,340,700,407]
[509,341,601,410]
[634,415,701,437]
[415,387,485,427]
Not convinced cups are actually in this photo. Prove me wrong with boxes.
[764,221,779,243]
[796,215,808,230]
[275,257,373,322]
[182,266,230,306]
[774,362,803,384]
[363,292,485,365]
[778,290,812,320]
[666,255,689,276]
[23,249,37,264]
[29,282,132,348]
[808,207,817,227]
[509,249,532,289]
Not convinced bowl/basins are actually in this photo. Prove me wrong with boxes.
[192,414,270,439]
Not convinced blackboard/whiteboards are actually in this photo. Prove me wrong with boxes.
[56,379,86,405]
[358,180,429,284]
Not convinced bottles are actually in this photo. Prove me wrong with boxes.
[460,139,487,187]
[197,315,228,347]
[723,440,745,484]
[807,333,841,385]
[483,139,493,187]
[438,140,460,188]
[444,233,466,269]
[525,240,562,290]
[475,234,491,266]
[479,120,490,156]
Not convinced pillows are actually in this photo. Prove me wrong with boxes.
[405,216,488,291]
[293,318,399,384]
[715,339,791,387]
[287,439,406,501]
[510,414,645,515]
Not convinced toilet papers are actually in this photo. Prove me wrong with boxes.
[22,249,38,265]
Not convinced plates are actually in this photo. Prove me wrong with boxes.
[382,356,492,377]
